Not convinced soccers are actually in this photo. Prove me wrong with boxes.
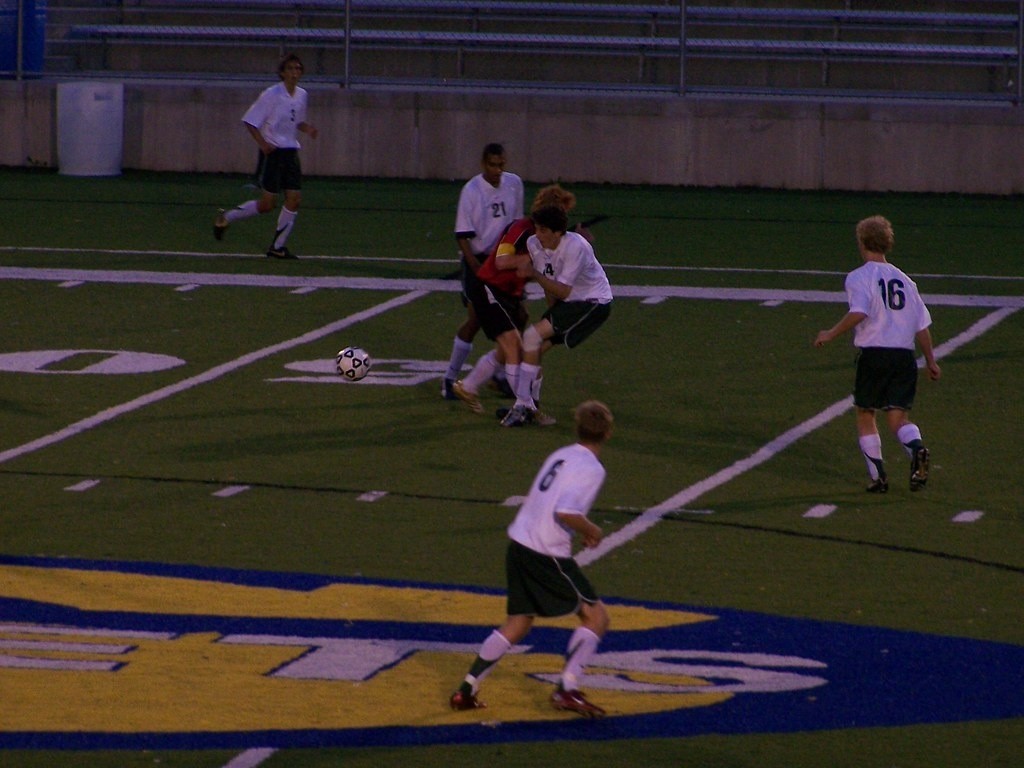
[335,346,371,381]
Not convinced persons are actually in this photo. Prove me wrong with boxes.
[213,54,318,259]
[497,204,614,428]
[453,185,596,424]
[450,400,613,718]
[441,143,525,399]
[814,215,941,492]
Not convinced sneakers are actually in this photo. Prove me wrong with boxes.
[496,399,558,428]
[549,690,606,720]
[266,244,299,261]
[866,480,894,494]
[450,691,488,712]
[909,446,931,492]
[214,208,228,241]
[492,374,517,400]
[440,374,456,399]
[453,380,486,418]
